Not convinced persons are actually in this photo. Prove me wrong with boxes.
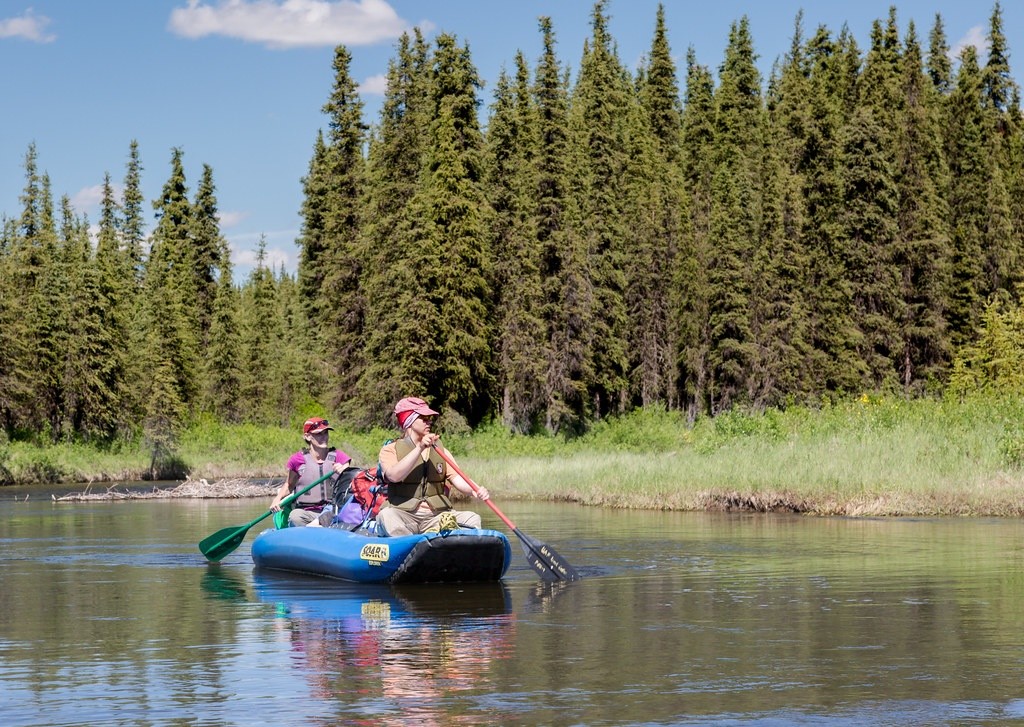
[270,417,352,529]
[376,397,489,537]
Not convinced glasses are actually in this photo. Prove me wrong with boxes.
[419,416,432,421]
[307,420,328,433]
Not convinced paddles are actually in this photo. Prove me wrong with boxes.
[430,440,580,583]
[198,470,335,562]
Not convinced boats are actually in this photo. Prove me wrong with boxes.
[251,527,512,585]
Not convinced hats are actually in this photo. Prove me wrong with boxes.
[394,397,439,415]
[398,410,420,430]
[304,417,335,434]
